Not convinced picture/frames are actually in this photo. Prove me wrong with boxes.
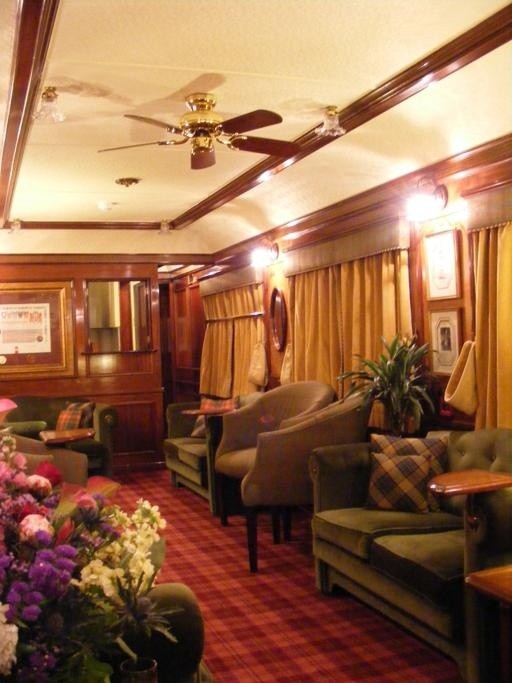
[0,278,77,381]
[419,227,462,301]
[427,307,463,377]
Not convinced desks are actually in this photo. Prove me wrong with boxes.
[464,564,512,682]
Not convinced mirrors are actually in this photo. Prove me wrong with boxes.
[80,276,158,355]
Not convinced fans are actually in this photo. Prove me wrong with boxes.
[96,92,301,169]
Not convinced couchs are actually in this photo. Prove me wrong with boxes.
[163,391,263,515]
[307,426,512,682]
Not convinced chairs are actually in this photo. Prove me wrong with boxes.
[206,381,335,545]
[239,392,374,572]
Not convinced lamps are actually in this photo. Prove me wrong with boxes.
[315,106,346,137]
[31,86,65,125]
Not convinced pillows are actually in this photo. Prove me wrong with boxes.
[57,400,96,432]
[190,395,239,437]
[368,432,448,513]
